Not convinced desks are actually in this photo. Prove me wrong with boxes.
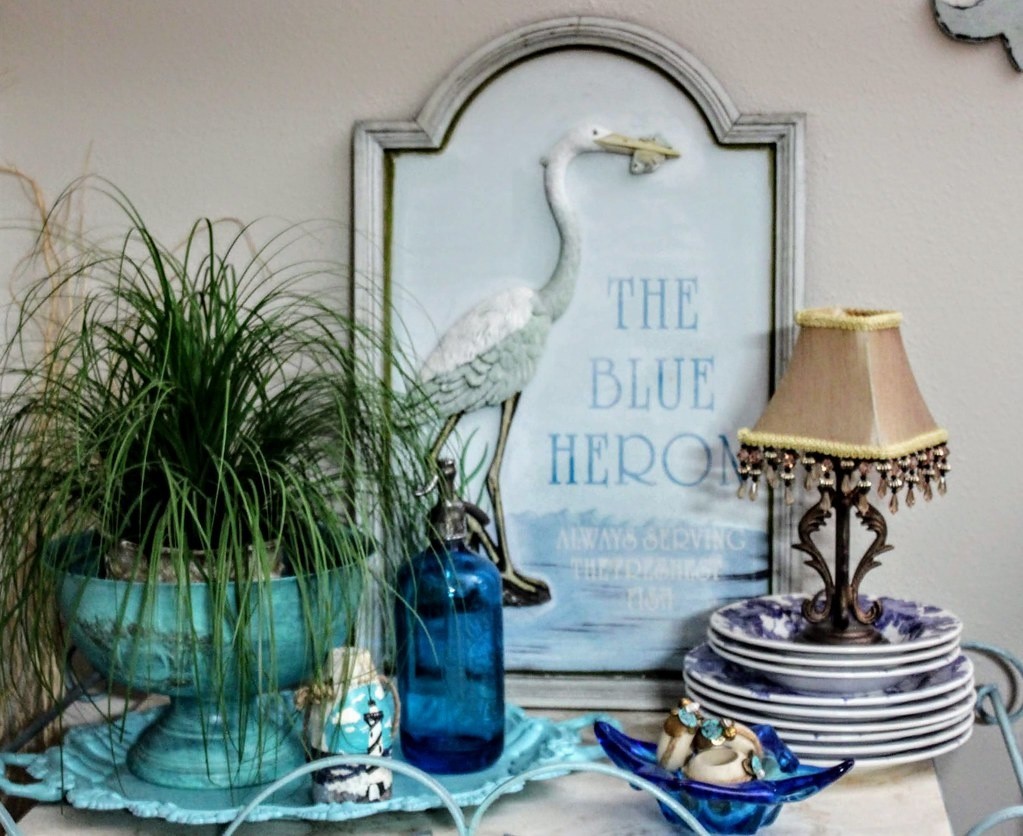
[0,638,1023,836]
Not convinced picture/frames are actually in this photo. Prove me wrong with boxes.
[351,15,806,712]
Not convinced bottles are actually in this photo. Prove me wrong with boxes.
[302,648,393,802]
[396,456,504,775]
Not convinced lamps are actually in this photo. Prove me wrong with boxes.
[736,305,952,643]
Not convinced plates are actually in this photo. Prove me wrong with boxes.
[681,591,977,770]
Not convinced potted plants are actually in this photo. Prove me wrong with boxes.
[1,138,478,790]
